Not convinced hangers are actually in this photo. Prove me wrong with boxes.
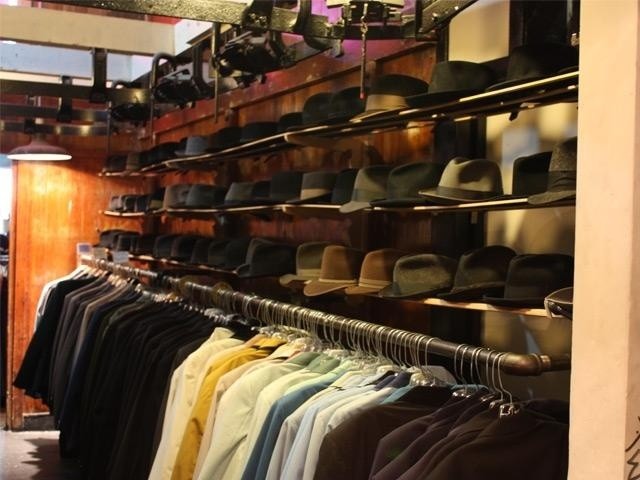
[58,256,527,420]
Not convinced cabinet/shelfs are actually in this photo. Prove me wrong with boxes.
[97,70,578,318]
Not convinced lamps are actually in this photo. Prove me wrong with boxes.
[6,94,73,161]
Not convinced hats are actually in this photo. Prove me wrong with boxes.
[278,48,575,316]
[99,122,294,278]
